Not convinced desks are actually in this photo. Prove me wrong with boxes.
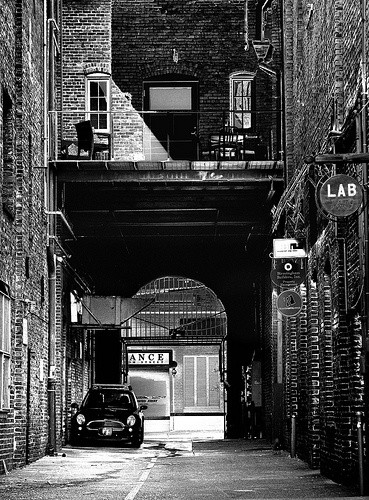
[209,135,262,160]
[63,137,109,159]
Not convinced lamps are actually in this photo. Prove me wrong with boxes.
[172,368,177,377]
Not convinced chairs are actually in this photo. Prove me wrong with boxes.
[209,126,238,160]
[75,120,112,160]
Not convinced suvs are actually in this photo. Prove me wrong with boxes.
[70,383,149,450]
